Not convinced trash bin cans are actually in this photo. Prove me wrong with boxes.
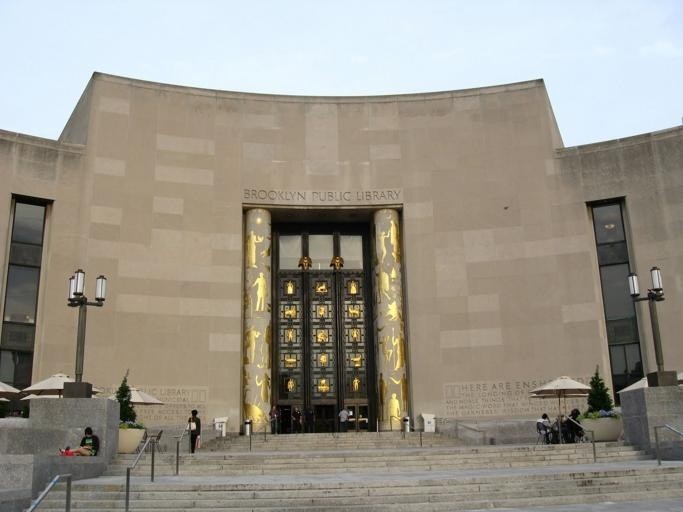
[403,416,410,432]
[243,420,252,436]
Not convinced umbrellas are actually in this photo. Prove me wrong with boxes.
[616,377,648,394]
[529,375,592,443]
[528,393,590,419]
[108,385,164,407]
[0,372,102,403]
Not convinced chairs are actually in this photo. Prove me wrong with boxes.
[137,430,163,452]
[536,420,554,445]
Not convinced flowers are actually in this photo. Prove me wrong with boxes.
[577,408,621,419]
[118,419,145,429]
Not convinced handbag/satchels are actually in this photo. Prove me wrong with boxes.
[184,422,196,431]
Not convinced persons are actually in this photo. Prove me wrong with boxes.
[188,410,201,454]
[536,409,580,444]
[338,409,349,432]
[304,408,315,433]
[269,405,279,434]
[291,407,302,433]
[59,427,99,456]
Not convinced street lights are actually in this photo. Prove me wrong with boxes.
[626,265,666,371]
[67,269,108,382]
